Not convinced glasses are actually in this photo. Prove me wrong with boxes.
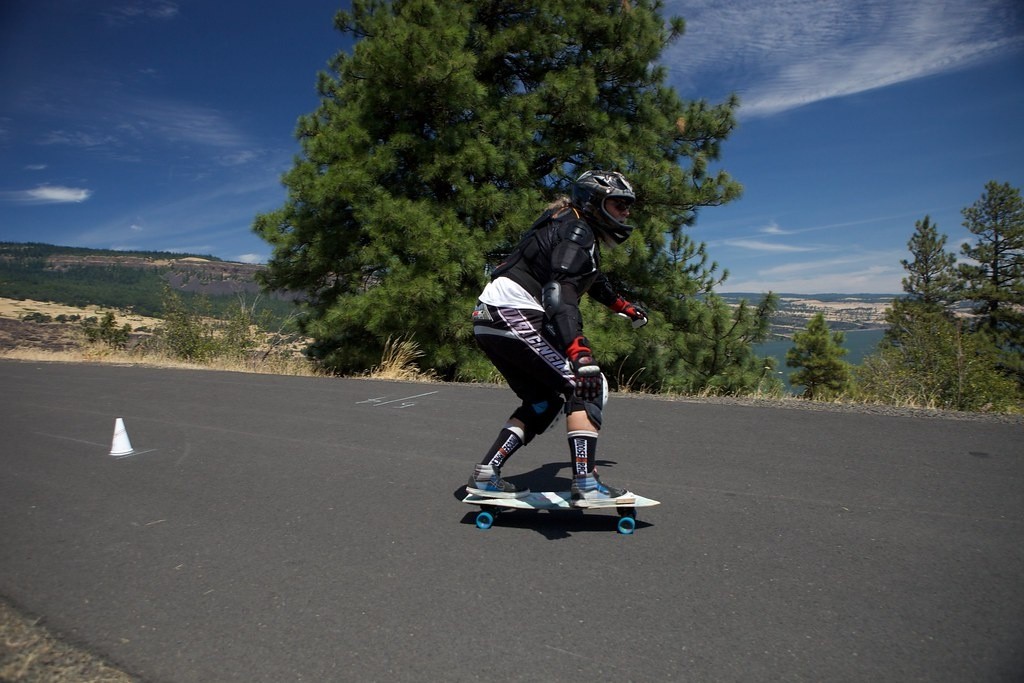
[608,198,631,210]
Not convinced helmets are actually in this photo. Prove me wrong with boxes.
[572,169,635,244]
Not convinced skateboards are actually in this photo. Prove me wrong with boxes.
[462,491,661,534]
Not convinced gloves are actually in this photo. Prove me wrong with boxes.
[610,293,648,330]
[566,336,603,402]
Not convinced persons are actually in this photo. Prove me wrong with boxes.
[464,170,649,506]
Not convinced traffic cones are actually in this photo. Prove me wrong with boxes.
[108,418,135,456]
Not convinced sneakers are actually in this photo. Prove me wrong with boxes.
[466,463,530,499]
[571,468,636,505]
